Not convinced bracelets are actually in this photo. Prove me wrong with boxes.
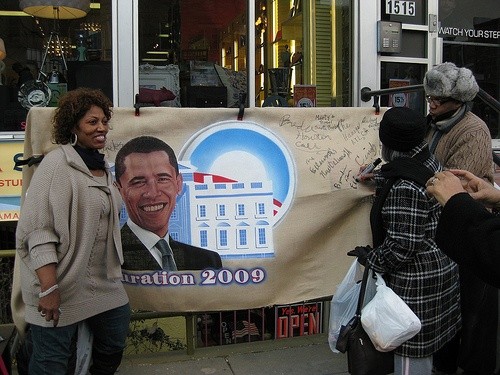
[39,284,59,298]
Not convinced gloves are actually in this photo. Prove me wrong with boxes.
[346,244,374,265]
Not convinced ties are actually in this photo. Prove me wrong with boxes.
[155,241,177,273]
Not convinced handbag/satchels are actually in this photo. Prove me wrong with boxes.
[328,258,422,375]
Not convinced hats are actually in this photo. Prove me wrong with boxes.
[423,62,480,102]
[378,106,426,153]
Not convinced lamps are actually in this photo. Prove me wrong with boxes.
[19,0,90,33]
[43,19,73,58]
[78,15,103,34]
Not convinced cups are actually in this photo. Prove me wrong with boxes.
[268,68,295,106]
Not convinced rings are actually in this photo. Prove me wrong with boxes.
[433,178,439,185]
[58,308,61,312]
[41,311,47,317]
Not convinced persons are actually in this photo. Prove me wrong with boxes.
[12,62,34,89]
[423,62,494,212]
[425,169,500,289]
[347,107,462,375]
[114,136,223,271]
[16,87,131,375]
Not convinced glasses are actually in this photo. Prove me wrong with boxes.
[427,94,455,107]
